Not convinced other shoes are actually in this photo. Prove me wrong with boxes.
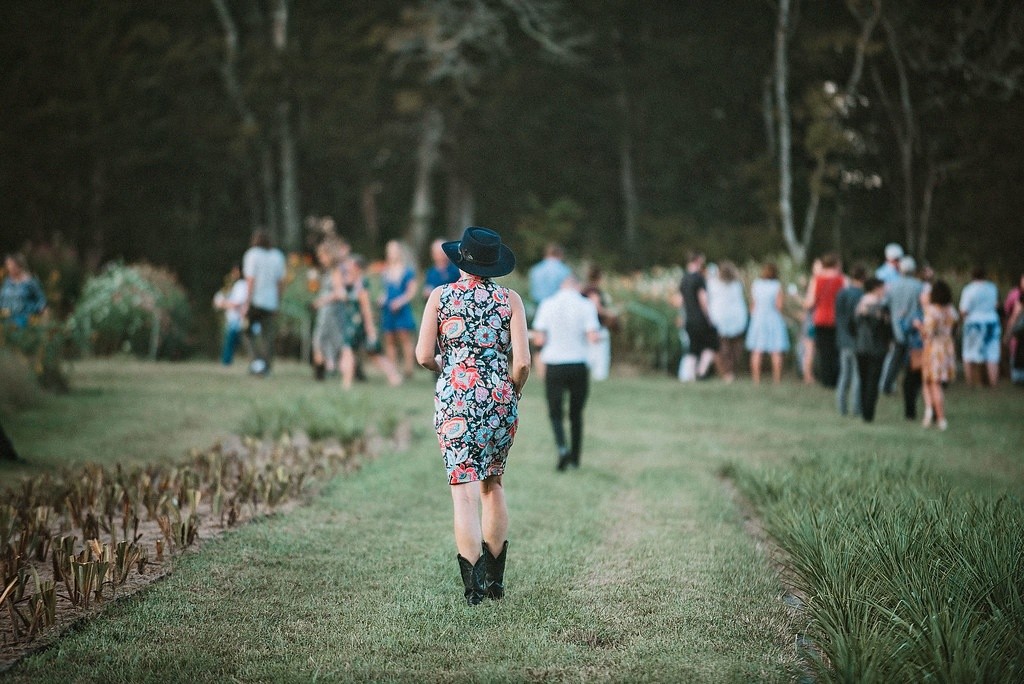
[936,416,948,432]
[922,407,934,427]
[557,446,580,473]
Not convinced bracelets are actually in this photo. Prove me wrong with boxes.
[517,392,522,400]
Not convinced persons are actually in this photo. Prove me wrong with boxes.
[531,277,599,470]
[0,252,48,356]
[528,239,615,382]
[55,251,89,315]
[415,226,531,608]
[674,243,1024,431]
[213,226,460,383]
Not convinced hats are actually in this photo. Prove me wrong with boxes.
[898,256,916,274]
[884,243,904,262]
[439,225,516,278]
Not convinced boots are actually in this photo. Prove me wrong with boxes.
[456,554,485,606]
[483,540,510,599]
